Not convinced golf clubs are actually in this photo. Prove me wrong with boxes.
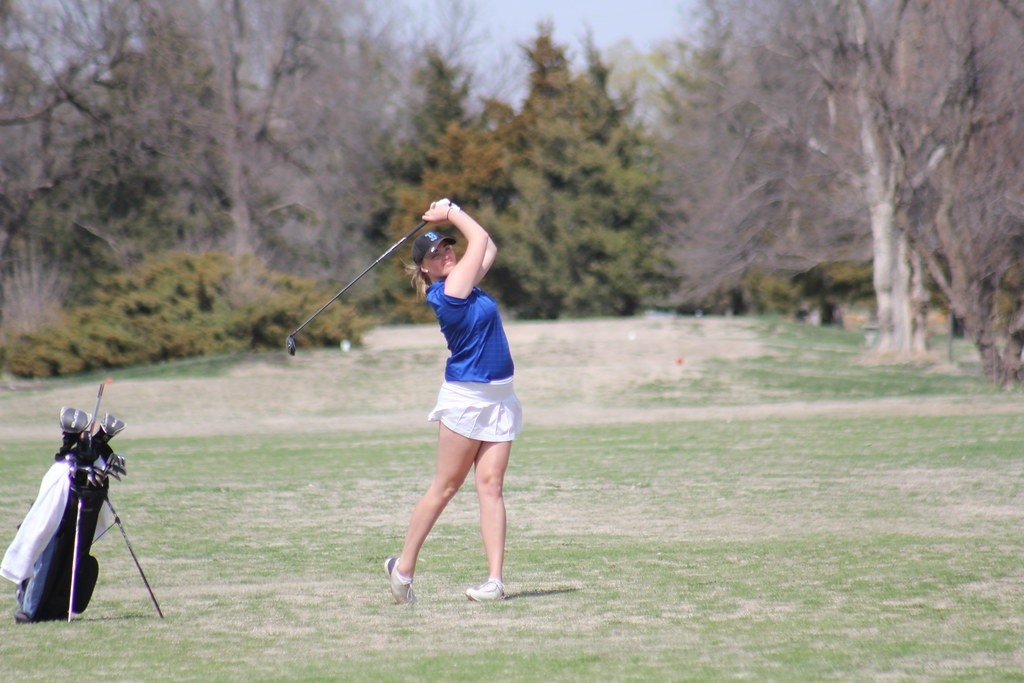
[285,219,429,357]
[55,405,129,490]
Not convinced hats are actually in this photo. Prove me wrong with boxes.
[412,231,456,268]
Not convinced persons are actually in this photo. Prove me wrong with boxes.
[385,196,520,610]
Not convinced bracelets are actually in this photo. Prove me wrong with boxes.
[446,208,455,223]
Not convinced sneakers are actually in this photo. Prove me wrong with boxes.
[465,578,508,602]
[384,558,416,604]
